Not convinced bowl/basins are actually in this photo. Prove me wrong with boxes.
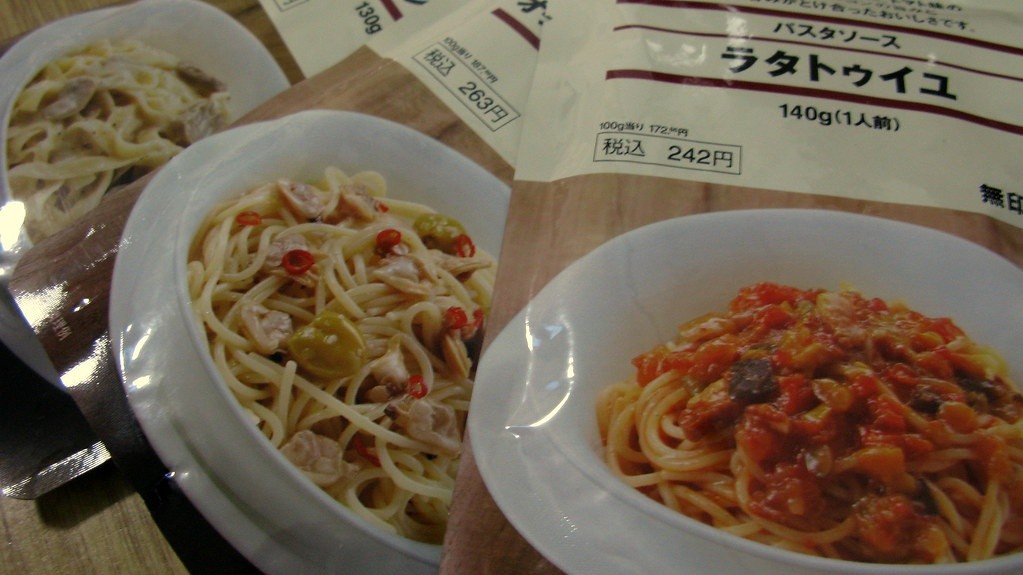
[174,108,511,568]
[3,0,294,397]
[520,209,1023,574]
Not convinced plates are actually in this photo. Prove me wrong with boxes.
[108,110,445,575]
[469,310,859,575]
[0,6,133,394]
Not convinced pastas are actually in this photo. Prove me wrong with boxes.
[188,162,496,549]
[5,34,240,245]
[597,281,1023,566]
